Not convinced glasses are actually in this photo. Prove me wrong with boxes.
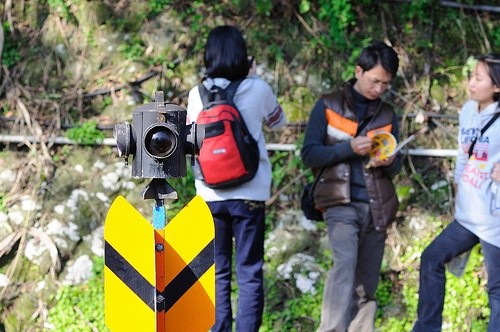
[362,71,392,90]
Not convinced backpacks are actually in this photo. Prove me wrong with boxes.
[194,78,260,189]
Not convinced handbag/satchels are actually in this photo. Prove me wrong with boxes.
[300,184,323,221]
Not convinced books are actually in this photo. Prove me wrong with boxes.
[363,130,416,170]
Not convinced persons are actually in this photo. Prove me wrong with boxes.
[186,25,287,332]
[410,52,500,332]
[299,40,403,332]
